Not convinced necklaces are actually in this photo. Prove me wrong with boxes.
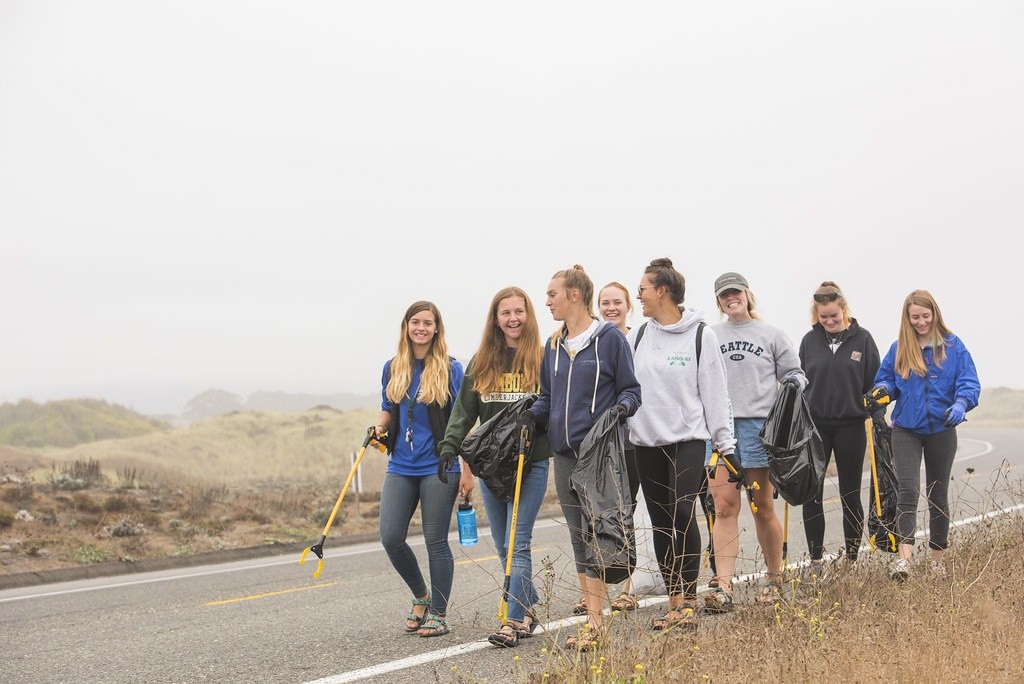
[826,332,843,350]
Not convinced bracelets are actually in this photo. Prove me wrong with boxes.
[376,424,386,432]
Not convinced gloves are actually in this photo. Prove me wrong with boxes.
[945,403,966,429]
[515,411,536,442]
[782,377,801,389]
[861,382,892,411]
[725,454,748,489]
[872,415,888,434]
[438,453,455,484]
[611,405,627,424]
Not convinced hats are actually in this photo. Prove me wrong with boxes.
[715,272,749,297]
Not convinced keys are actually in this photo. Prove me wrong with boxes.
[408,433,414,451]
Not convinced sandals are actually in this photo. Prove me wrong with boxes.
[417,613,450,637]
[404,589,431,632]
[517,608,539,639]
[708,573,719,588]
[704,587,734,610]
[565,621,608,652]
[488,621,520,648]
[573,595,588,613]
[611,592,639,610]
[652,603,698,630]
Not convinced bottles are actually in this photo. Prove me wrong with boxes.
[457,497,478,546]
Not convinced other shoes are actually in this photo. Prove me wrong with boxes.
[931,561,948,580]
[891,559,915,582]
[759,573,783,602]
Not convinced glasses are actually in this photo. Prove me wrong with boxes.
[813,293,843,302]
[638,285,668,295]
[720,289,742,298]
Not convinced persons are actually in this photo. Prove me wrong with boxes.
[863,291,980,582]
[799,281,882,581]
[698,272,808,612]
[368,301,474,636]
[437,287,551,646]
[626,259,748,632]
[514,265,642,652]
